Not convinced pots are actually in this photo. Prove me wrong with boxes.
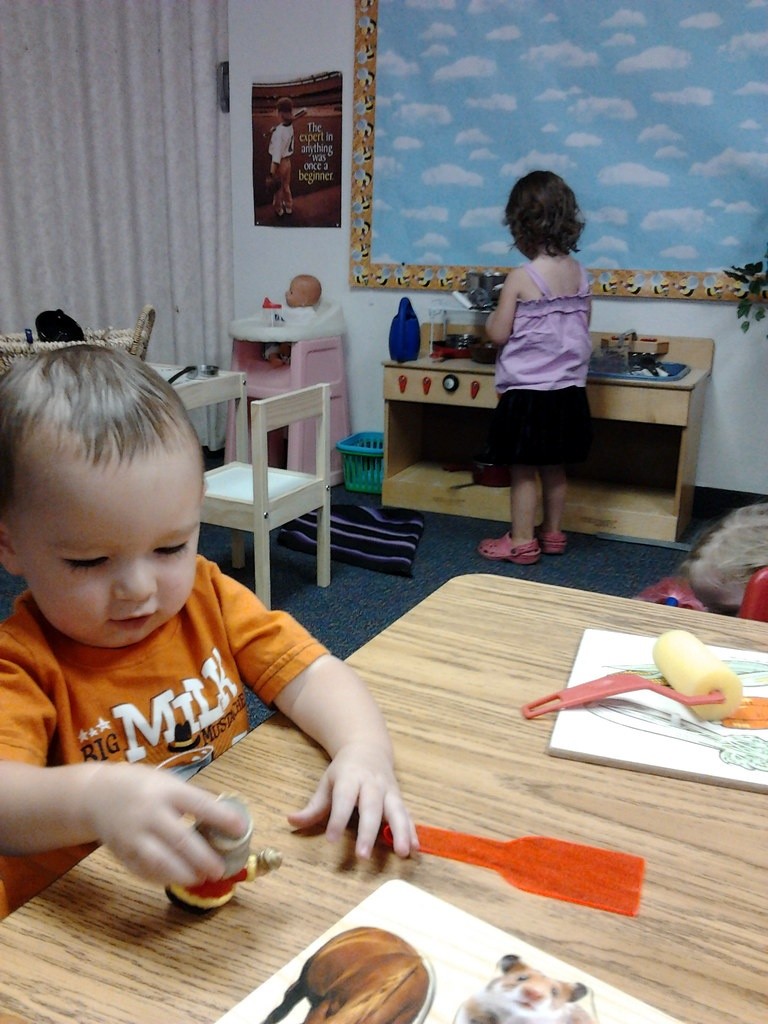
[430,340,469,362]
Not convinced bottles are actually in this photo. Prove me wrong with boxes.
[261,297,283,328]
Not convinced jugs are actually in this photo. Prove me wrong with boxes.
[389,297,421,361]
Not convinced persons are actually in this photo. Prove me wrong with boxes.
[263,274,321,368]
[682,503,768,617]
[267,97,295,216]
[0,343,420,922]
[478,171,592,564]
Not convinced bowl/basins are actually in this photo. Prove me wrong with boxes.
[464,271,506,296]
[471,343,499,363]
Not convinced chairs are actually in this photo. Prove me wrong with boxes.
[223,299,351,487]
[199,383,332,610]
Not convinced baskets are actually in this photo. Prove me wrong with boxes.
[337,432,384,494]
[0,305,156,377]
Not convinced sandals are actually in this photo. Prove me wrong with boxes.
[478,531,541,564]
[534,526,567,553]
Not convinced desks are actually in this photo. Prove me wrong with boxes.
[145,362,250,569]
[1,572,768,1024]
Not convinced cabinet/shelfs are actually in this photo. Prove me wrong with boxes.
[381,322,716,550]
